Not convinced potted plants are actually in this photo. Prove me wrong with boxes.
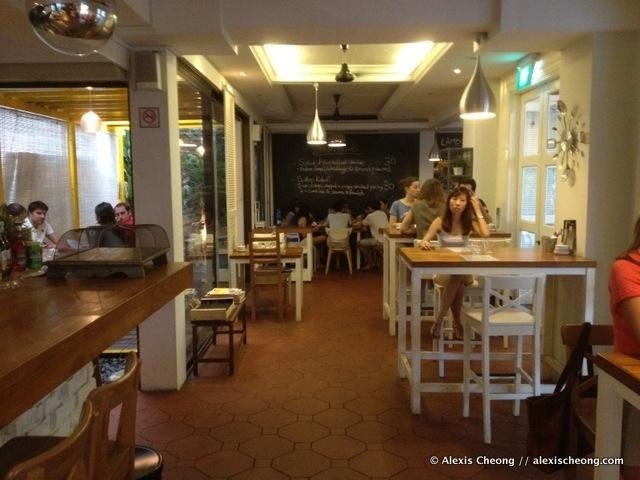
[451,157,466,176]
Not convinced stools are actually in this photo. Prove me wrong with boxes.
[193,299,247,376]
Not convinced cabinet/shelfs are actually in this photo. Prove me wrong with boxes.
[437,147,475,197]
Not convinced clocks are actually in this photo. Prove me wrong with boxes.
[544,100,587,183]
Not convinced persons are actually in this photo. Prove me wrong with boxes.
[0,202,27,233]
[608,213,640,358]
[22,200,70,252]
[95,203,134,249]
[279,175,490,342]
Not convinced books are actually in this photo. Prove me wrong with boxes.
[204,287,247,303]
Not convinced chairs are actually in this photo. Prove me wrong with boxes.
[379,227,598,444]
[1,350,140,479]
[229,215,379,323]
[3,403,95,480]
[561,321,640,480]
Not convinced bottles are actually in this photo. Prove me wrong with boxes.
[0,219,27,279]
[276,208,281,227]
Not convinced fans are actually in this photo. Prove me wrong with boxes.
[308,44,391,83]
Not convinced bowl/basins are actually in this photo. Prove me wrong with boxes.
[443,238,464,247]
[468,238,490,256]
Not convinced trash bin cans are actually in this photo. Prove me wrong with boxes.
[132,444,165,480]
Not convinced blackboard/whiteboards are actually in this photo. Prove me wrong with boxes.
[261,127,422,230]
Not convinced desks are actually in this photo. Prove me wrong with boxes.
[593,352,640,480]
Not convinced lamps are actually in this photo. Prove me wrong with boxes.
[79,85,103,136]
[458,34,497,121]
[427,125,444,162]
[23,0,117,56]
[306,82,327,146]
[327,93,348,148]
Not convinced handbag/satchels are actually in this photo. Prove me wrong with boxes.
[525,373,598,473]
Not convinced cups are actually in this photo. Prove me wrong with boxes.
[542,238,558,253]
[238,246,245,253]
